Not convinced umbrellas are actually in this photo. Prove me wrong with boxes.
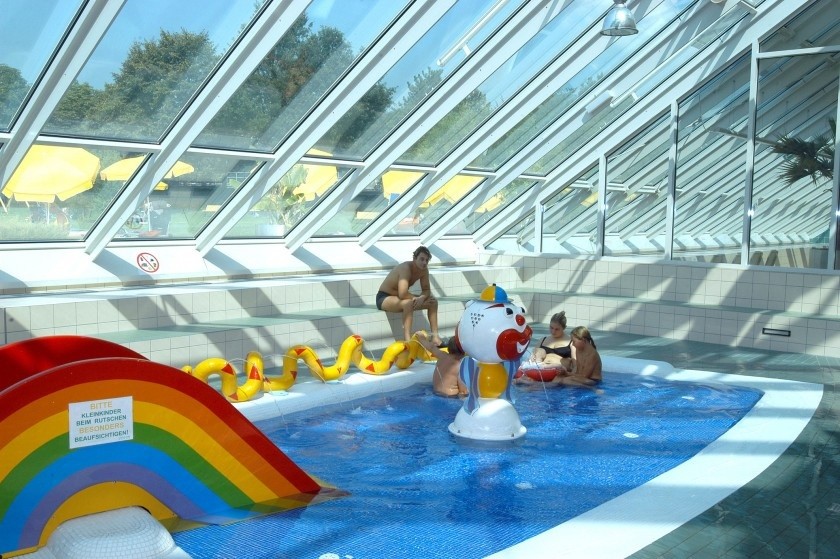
[3,143,102,224]
[382,169,506,213]
[100,155,196,240]
[562,186,638,209]
[251,148,339,203]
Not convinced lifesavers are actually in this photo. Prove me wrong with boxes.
[513,369,559,382]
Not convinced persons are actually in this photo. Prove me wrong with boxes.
[510,347,547,386]
[524,311,578,392]
[415,331,470,398]
[376,245,449,348]
[551,326,605,395]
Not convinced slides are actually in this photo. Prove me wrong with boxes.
[0,335,351,559]
